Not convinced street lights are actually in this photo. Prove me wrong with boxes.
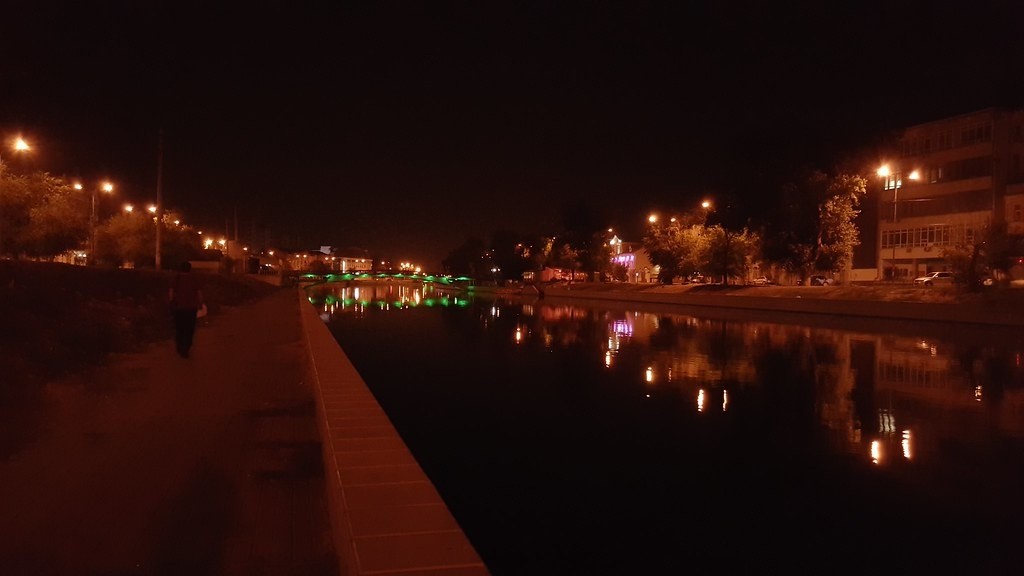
[876,162,921,290]
[636,272,639,284]
[74,180,113,266]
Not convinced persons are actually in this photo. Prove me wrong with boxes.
[167,262,202,358]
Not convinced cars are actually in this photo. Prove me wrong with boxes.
[797,274,833,287]
[752,276,775,286]
[913,271,955,287]
